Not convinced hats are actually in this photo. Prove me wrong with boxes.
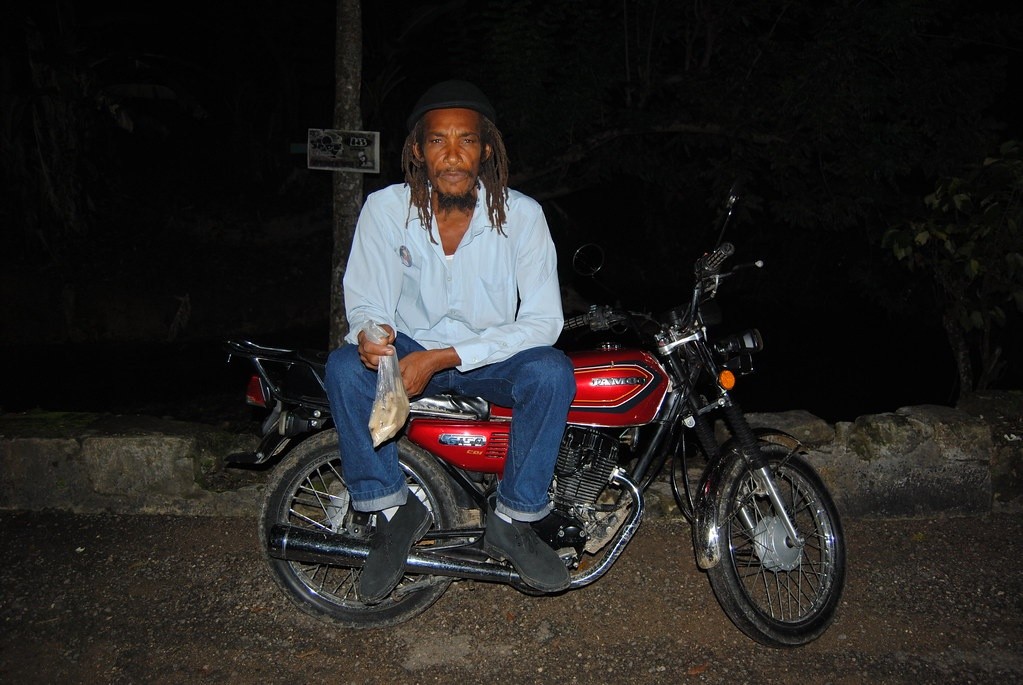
[407,79,495,133]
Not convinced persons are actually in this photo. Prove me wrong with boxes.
[325,81,576,605]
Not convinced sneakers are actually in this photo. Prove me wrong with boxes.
[484,495,571,592]
[358,488,433,603]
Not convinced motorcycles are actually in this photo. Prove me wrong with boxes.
[220,185,844,646]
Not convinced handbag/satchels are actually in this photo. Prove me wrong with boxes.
[360,320,411,448]
